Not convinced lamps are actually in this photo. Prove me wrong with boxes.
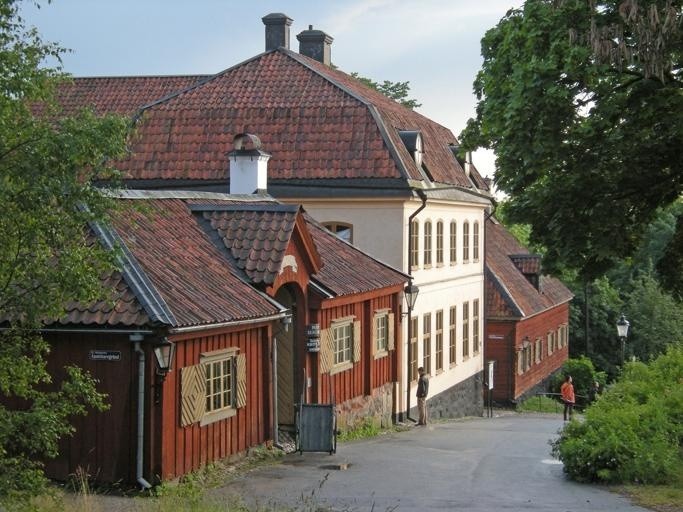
[514,335,530,356]
[151,336,174,396]
[398,279,419,325]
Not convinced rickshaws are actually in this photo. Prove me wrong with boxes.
[291,366,341,455]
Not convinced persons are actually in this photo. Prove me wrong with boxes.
[561,375,574,420]
[415,366,428,426]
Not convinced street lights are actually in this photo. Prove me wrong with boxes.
[616,310,630,380]
